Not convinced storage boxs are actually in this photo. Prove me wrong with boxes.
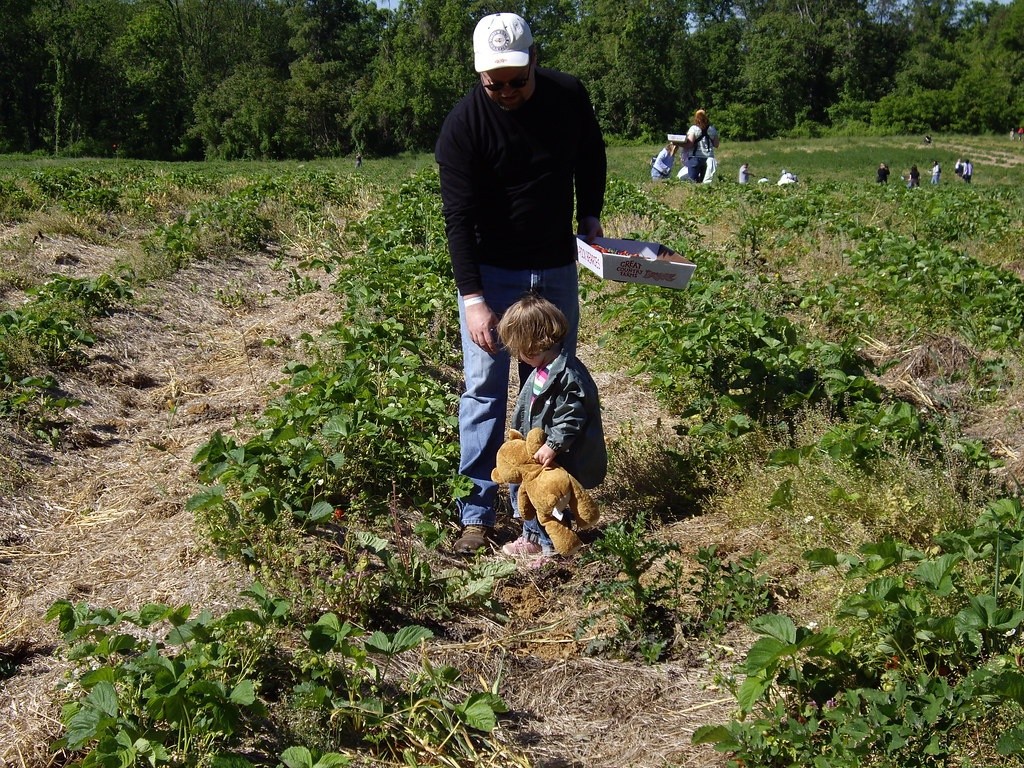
[576,235,697,291]
[667,134,687,142]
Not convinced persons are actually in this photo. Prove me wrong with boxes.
[876,163,891,183]
[955,158,973,183]
[682,109,721,182]
[1009,128,1015,140]
[650,142,679,181]
[929,160,941,184]
[738,162,756,183]
[926,134,933,143]
[758,176,769,183]
[778,169,799,185]
[1018,128,1023,141]
[498,295,609,566]
[907,164,921,188]
[355,153,363,169]
[434,12,608,551]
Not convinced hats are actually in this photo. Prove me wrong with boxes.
[472,12,534,72]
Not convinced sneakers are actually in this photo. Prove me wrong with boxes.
[453,524,494,552]
[504,536,543,555]
[527,558,548,568]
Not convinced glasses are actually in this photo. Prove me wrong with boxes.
[480,64,532,91]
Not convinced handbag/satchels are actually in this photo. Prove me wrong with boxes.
[684,157,698,167]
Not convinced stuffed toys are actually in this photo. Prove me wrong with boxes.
[491,427,600,558]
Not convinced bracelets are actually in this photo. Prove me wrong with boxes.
[464,296,485,307]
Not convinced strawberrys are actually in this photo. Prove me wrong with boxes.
[336,509,342,517]
[588,242,638,257]
